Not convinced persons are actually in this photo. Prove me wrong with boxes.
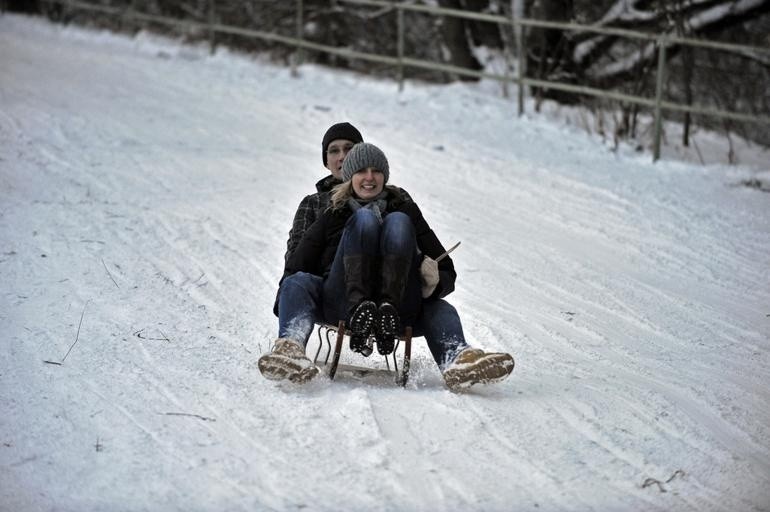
[253,121,516,393]
[292,138,456,353]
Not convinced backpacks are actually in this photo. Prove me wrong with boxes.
[419,255,440,300]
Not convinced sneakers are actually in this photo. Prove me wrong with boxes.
[372,298,400,356]
[442,345,514,392]
[257,336,321,385]
[348,299,378,355]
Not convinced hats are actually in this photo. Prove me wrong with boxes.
[321,122,363,167]
[342,142,389,183]
